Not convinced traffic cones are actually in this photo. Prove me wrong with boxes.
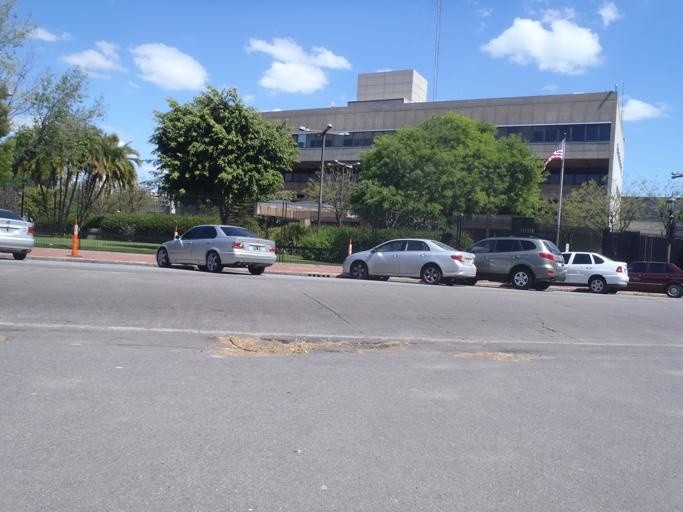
[173,226,179,240]
[348,239,353,257]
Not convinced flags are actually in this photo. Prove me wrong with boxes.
[540,139,563,173]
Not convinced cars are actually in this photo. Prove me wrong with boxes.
[343,238,477,285]
[462,236,567,291]
[0,208,36,260]
[619,261,683,297]
[154,225,277,276]
[555,252,629,294]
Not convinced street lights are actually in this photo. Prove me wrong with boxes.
[667,192,680,261]
[328,157,361,228]
[300,124,350,236]
